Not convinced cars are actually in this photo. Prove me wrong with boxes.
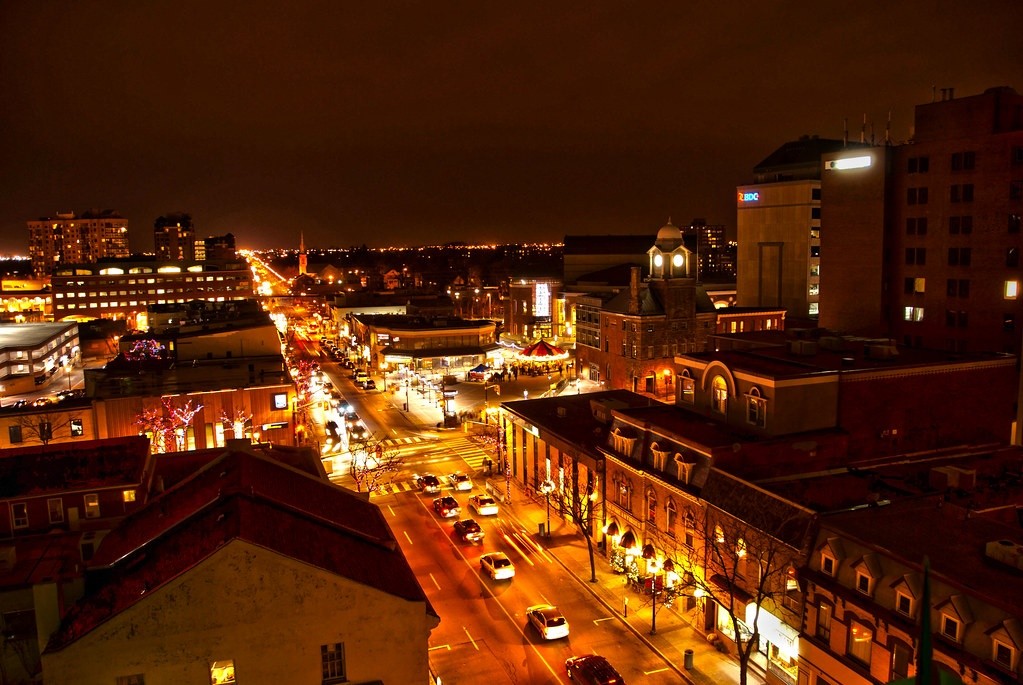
[432,495,461,519]
[468,493,499,516]
[526,603,571,640]
[416,474,442,495]
[564,654,626,685]
[452,519,485,543]
[478,552,516,581]
[310,333,378,442]
[447,472,473,492]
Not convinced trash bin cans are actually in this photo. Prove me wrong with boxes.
[403,403,407,410]
[683,649,693,670]
[539,523,545,537]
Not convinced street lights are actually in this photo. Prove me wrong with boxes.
[543,482,552,539]
[647,561,659,637]
[483,372,492,427]
